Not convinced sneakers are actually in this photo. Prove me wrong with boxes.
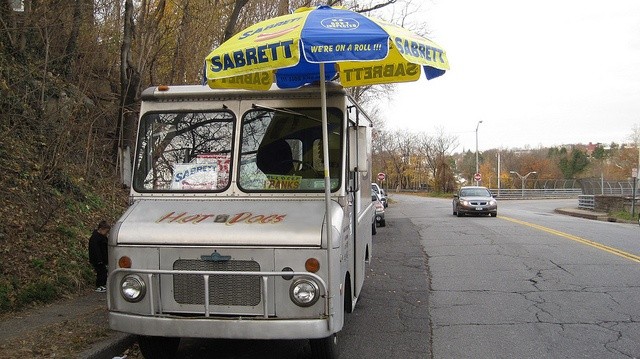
[99,284,108,290]
[94,286,105,292]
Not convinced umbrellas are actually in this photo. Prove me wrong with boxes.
[204,3,454,334]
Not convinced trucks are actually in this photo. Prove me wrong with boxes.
[106,83,373,358]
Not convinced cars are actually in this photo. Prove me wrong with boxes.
[372,188,385,227]
[453,186,497,217]
[372,183,381,202]
[380,189,388,207]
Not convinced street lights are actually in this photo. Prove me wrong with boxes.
[476,120,483,185]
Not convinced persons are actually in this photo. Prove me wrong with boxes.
[87,219,109,294]
